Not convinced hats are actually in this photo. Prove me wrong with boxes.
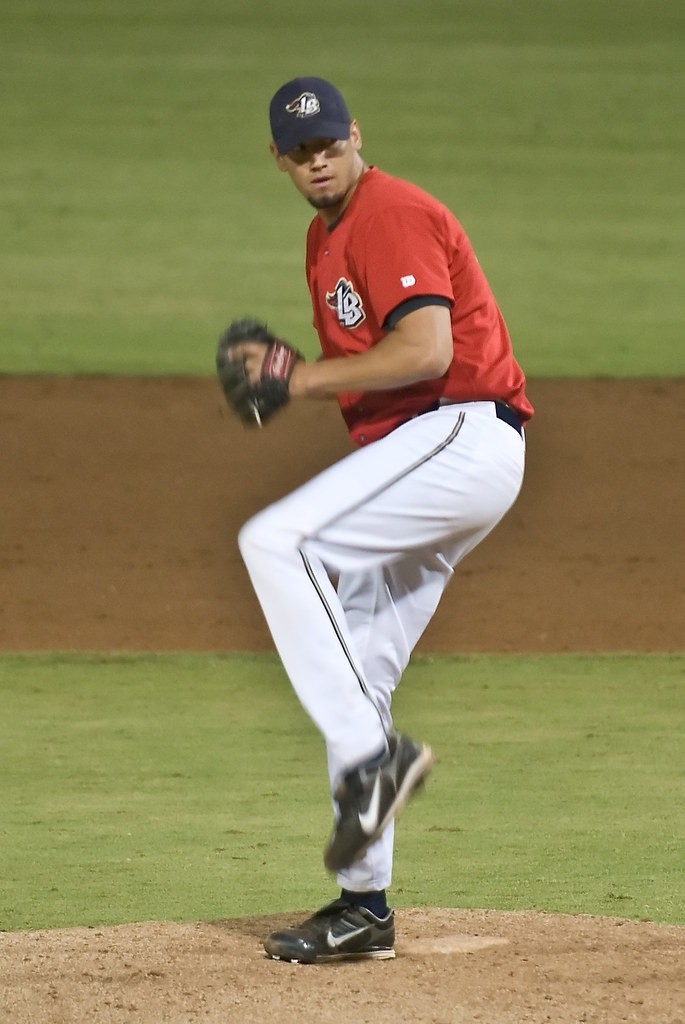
[269,77,350,155]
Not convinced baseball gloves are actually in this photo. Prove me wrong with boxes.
[216,319,308,429]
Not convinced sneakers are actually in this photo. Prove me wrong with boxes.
[264,888,395,963]
[324,727,436,871]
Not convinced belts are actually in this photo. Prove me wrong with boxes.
[395,403,526,441]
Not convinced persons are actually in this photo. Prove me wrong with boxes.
[228,79,536,966]
